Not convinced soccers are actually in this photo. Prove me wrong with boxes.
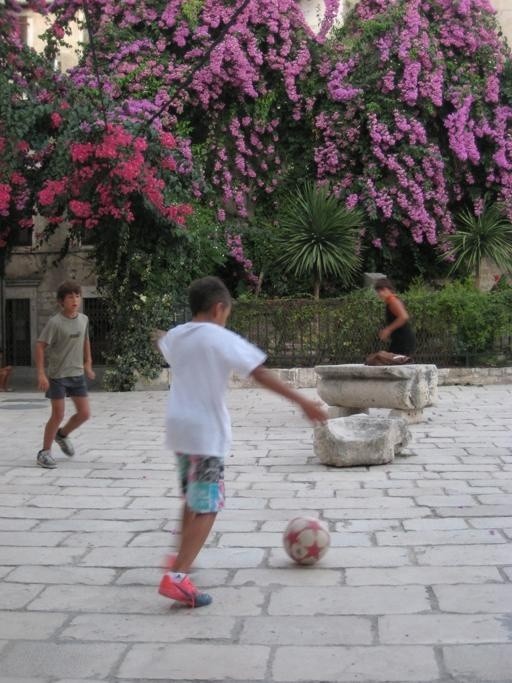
[283,516,330,566]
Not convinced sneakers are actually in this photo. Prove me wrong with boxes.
[159,552,213,609]
[36,448,58,469]
[55,428,75,456]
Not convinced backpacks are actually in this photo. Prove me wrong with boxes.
[366,351,414,366]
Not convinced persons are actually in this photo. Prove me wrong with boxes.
[374,277,418,355]
[149,276,333,608]
[35,279,96,469]
[0,351,13,392]
